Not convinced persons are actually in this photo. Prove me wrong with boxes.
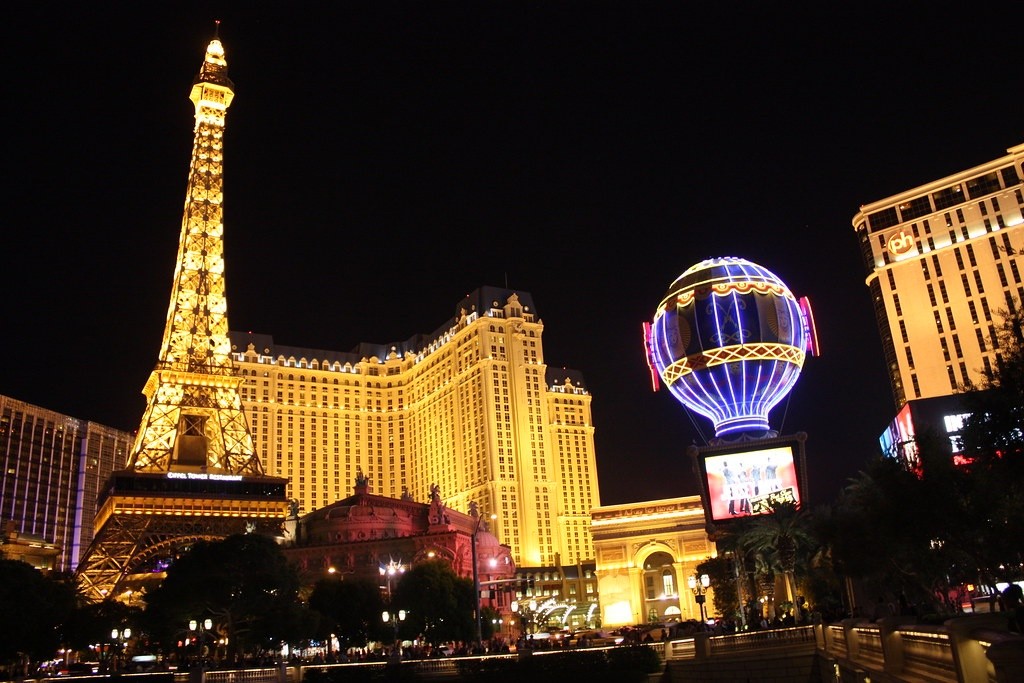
[1000,576,1024,633]
[0,596,808,683]
[722,456,779,516]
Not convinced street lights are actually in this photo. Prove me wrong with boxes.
[111,628,131,674]
[328,566,353,581]
[471,513,497,656]
[379,564,405,602]
[382,610,406,664]
[511,599,537,649]
[688,574,710,624]
[188,619,213,663]
